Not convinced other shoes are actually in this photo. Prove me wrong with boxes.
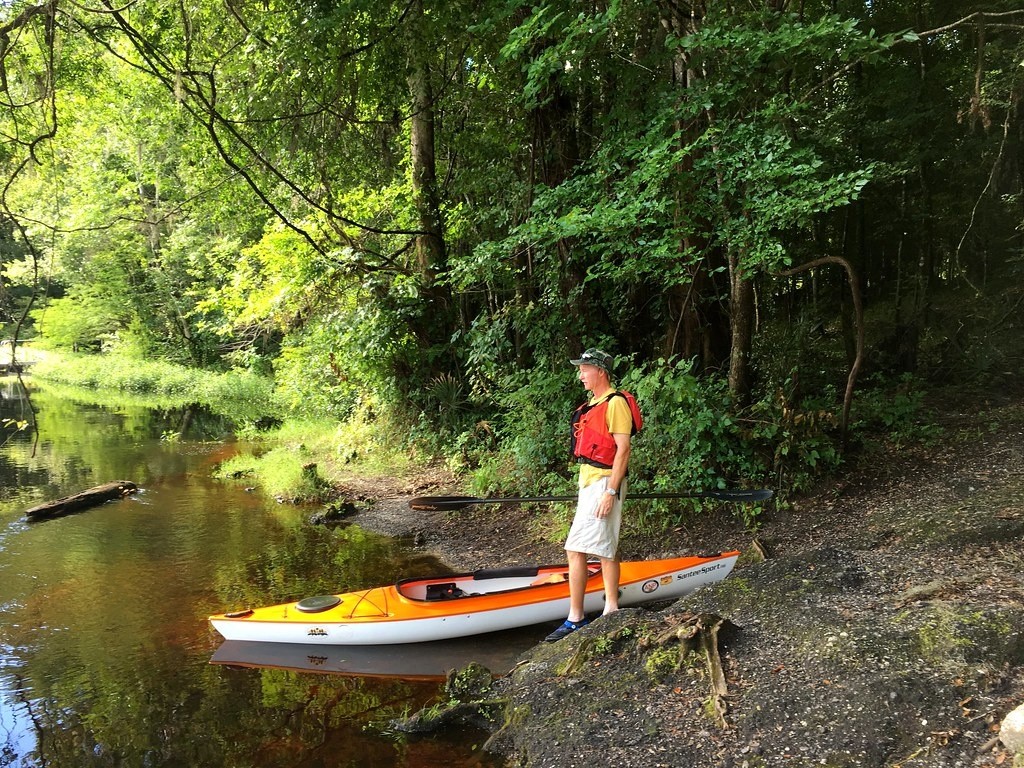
[546,619,590,641]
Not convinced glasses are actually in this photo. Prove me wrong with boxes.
[581,353,609,368]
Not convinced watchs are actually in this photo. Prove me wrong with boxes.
[606,488,618,495]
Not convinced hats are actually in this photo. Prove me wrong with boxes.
[569,347,614,374]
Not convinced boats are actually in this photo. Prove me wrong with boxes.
[207,550,742,676]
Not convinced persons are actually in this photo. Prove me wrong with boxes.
[545,347,633,642]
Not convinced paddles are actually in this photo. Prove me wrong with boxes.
[406,489,777,516]
